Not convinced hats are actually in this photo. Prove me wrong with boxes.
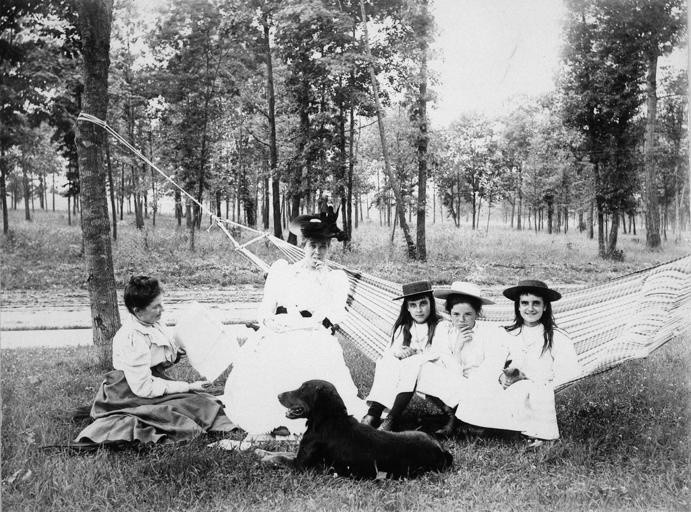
[433,279,499,307]
[392,278,436,302]
[287,208,341,242]
[502,278,563,304]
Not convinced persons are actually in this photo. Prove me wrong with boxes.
[417,280,497,440]
[75,275,239,446]
[454,278,562,450]
[357,277,443,431]
[225,207,370,439]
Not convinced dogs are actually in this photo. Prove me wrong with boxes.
[254,379,454,481]
